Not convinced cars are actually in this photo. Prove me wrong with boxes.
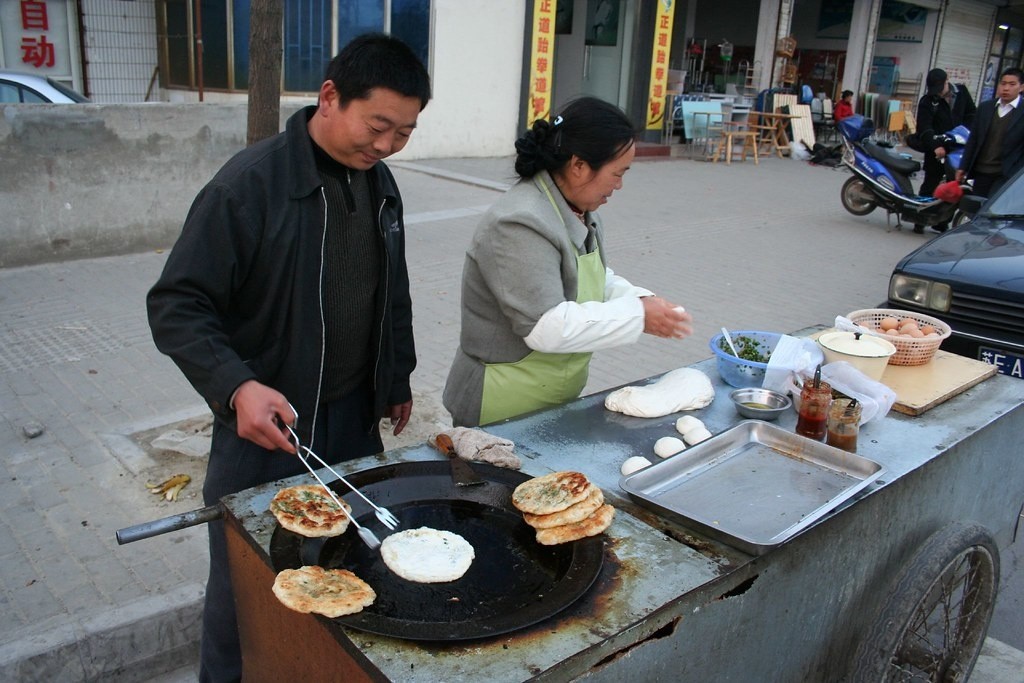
[1,68,92,103]
[872,164,1024,383]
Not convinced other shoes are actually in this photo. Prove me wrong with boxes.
[931,224,949,233]
[914,222,924,234]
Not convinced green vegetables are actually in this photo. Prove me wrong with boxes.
[721,335,771,375]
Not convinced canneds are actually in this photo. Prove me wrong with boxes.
[797,380,862,456]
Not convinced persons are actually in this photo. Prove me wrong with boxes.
[955,68,1024,197]
[834,90,853,125]
[441,97,691,429]
[147,33,430,683]
[912,68,976,234]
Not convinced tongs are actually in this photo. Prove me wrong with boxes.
[284,401,400,550]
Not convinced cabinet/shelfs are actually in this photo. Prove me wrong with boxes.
[734,60,763,97]
[890,69,923,117]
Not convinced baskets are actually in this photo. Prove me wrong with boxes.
[846,308,952,366]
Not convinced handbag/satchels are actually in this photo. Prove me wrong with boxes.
[933,180,963,202]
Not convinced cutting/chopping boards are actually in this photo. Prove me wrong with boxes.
[808,326,999,416]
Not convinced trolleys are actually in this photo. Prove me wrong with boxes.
[114,327,1024,683]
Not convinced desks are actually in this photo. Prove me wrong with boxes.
[860,91,906,143]
[691,112,730,156]
[680,99,724,159]
[749,112,803,160]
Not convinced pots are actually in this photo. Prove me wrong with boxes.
[269,460,605,641]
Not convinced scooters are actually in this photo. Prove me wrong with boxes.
[835,112,971,234]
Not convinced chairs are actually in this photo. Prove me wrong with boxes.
[802,85,813,103]
[823,97,837,124]
[811,99,836,142]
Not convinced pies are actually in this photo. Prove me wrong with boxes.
[512,471,615,545]
[272,565,377,618]
[380,526,475,582]
[270,484,352,537]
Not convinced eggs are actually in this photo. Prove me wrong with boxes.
[860,317,940,365]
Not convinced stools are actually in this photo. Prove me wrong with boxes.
[747,124,779,159]
[693,127,724,152]
[713,130,760,164]
[715,122,746,147]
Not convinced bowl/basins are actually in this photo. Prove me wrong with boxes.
[818,332,897,381]
[786,370,878,427]
[710,330,824,388]
[728,387,792,422]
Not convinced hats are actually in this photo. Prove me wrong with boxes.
[926,68,947,96]
